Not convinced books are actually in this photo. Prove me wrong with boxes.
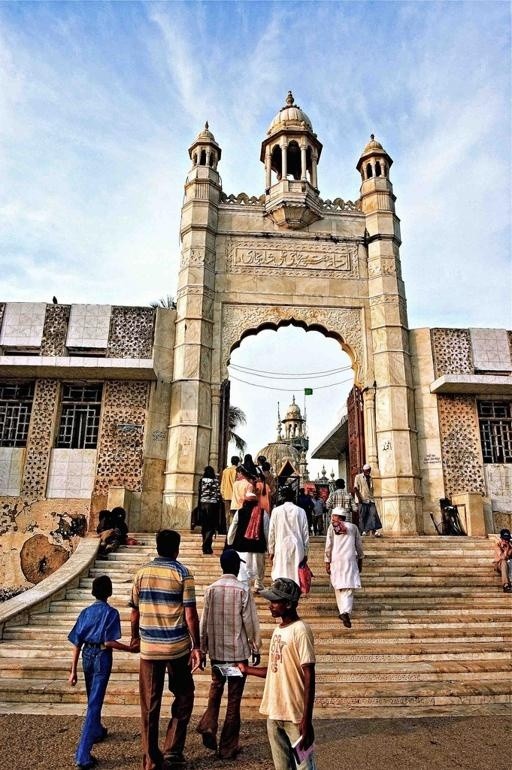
[210,662,244,678]
[291,734,315,766]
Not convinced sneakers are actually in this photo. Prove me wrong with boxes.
[202,728,217,750]
[79,757,97,769]
[338,614,352,628]
[164,750,186,764]
[94,727,107,742]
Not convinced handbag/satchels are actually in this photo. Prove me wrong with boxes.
[299,565,316,593]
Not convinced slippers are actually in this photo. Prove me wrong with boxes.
[372,531,382,536]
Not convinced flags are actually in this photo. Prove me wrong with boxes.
[304,388,313,396]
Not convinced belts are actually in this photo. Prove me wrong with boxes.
[83,642,103,648]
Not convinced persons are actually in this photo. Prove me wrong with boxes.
[324,507,363,628]
[195,550,262,758]
[235,577,317,770]
[195,453,384,556]
[490,529,512,594]
[227,493,270,592]
[127,530,201,770]
[269,488,310,596]
[67,576,140,770]
[96,506,129,561]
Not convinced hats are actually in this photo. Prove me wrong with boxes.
[246,492,257,496]
[500,529,511,539]
[221,550,246,565]
[332,508,346,517]
[363,464,370,470]
[259,578,302,604]
